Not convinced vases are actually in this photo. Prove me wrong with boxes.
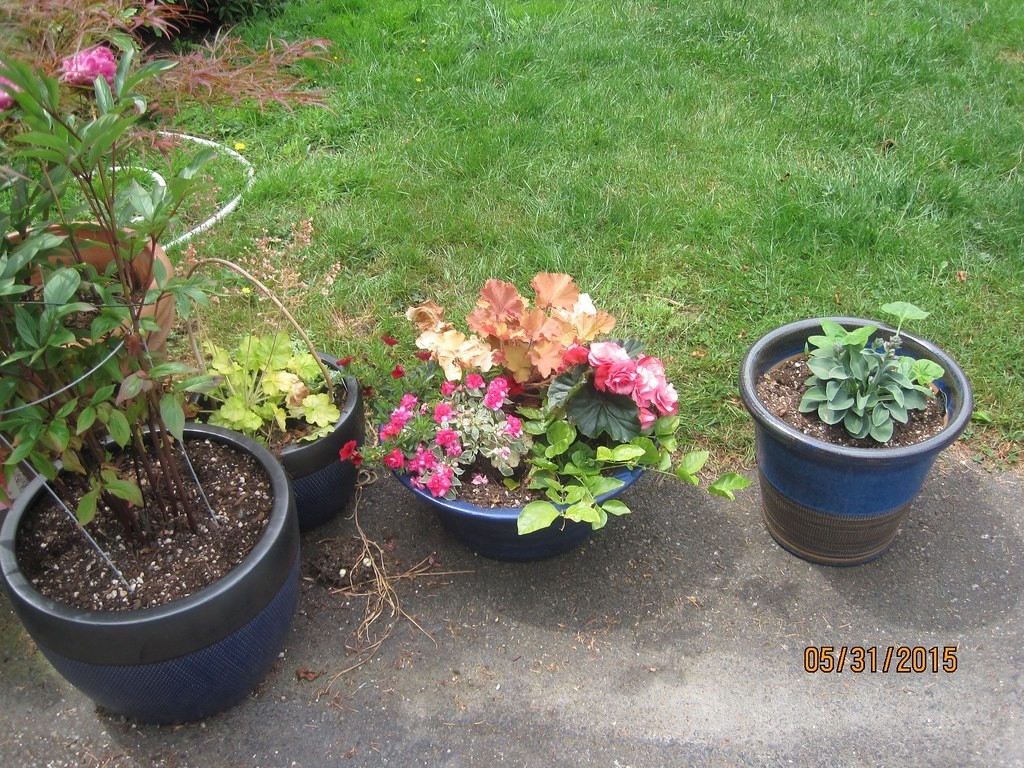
[380,372,646,562]
[0,220,175,459]
[0,423,304,724]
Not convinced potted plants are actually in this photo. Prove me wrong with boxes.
[739,300,975,567]
[187,352,364,530]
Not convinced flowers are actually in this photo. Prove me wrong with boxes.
[0,38,261,549]
[0,0,220,433]
[341,271,752,537]
